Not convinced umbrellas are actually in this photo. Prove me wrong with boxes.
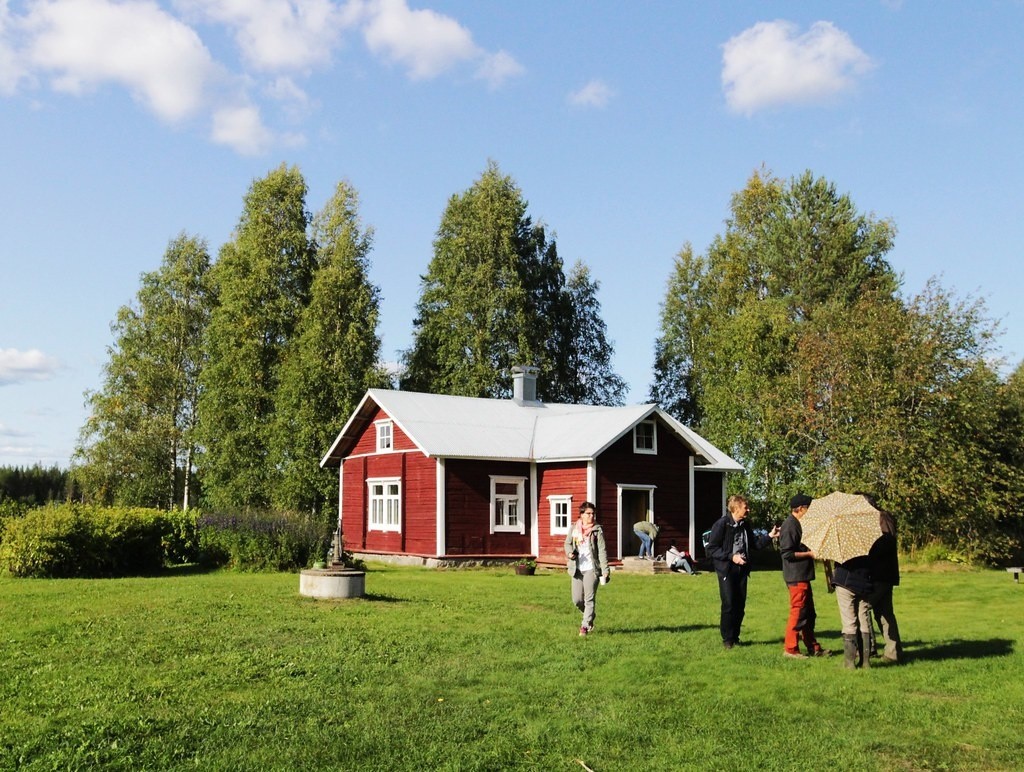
[798,490,883,565]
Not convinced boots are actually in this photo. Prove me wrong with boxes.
[843,633,858,669]
[855,632,871,668]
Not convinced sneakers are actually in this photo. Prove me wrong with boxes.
[587,613,596,632]
[579,626,588,636]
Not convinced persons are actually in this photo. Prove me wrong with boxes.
[633,521,661,560]
[564,501,611,637]
[708,495,781,649]
[831,493,904,669]
[779,493,832,658]
[665,540,702,576]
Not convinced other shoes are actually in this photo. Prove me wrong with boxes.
[808,648,833,656]
[878,656,904,663]
[690,571,702,577]
[869,650,878,658]
[734,637,743,644]
[639,557,646,560]
[723,640,731,650]
[647,556,656,561]
[783,651,810,659]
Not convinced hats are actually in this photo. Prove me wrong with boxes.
[791,493,815,507]
